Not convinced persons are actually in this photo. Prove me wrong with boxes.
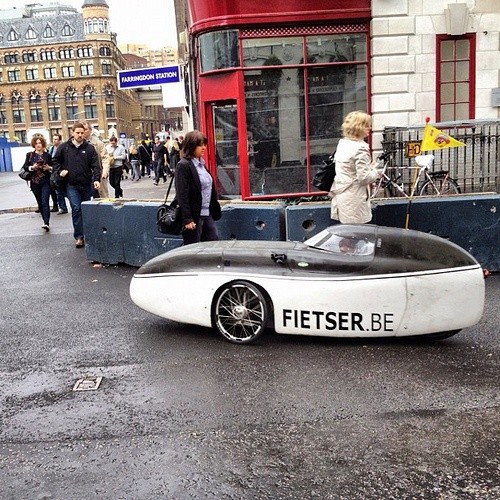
[23,136,55,231]
[326,110,389,227]
[107,137,126,197]
[53,123,103,247]
[46,134,65,211]
[172,130,223,246]
[78,122,109,200]
[121,122,184,186]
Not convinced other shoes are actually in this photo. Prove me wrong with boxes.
[35,209,41,212]
[50,206,58,212]
[42,225,49,231]
[76,239,84,248]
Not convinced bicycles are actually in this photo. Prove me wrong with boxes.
[367,140,463,199]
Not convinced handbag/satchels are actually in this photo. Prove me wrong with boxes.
[49,165,67,190]
[156,195,187,236]
[18,161,34,180]
[312,151,336,192]
